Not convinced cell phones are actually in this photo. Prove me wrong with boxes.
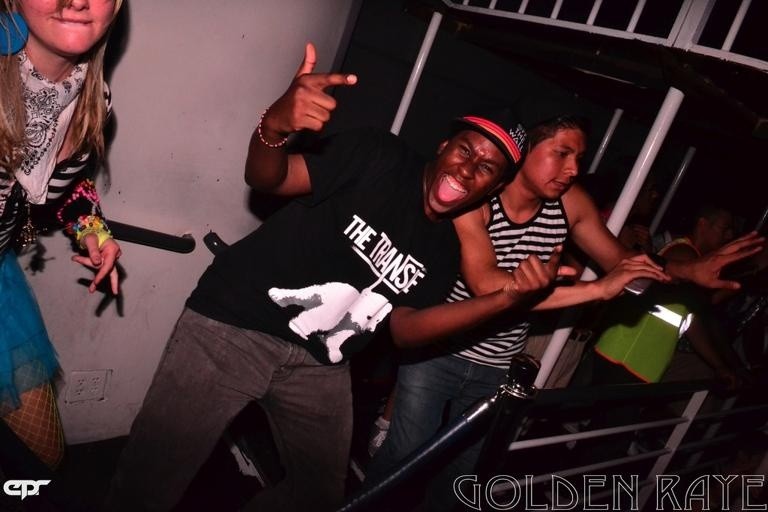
[626,256,670,294]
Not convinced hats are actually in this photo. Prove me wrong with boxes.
[449,100,529,178]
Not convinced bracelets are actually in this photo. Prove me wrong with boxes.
[649,252,670,276]
[51,180,99,225]
[70,215,112,239]
[258,106,291,149]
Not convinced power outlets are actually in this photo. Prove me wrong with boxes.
[66,371,107,404]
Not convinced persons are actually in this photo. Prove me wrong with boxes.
[0,0,122,467]
[577,154,766,390]
[124,42,576,468]
[370,100,766,508]
